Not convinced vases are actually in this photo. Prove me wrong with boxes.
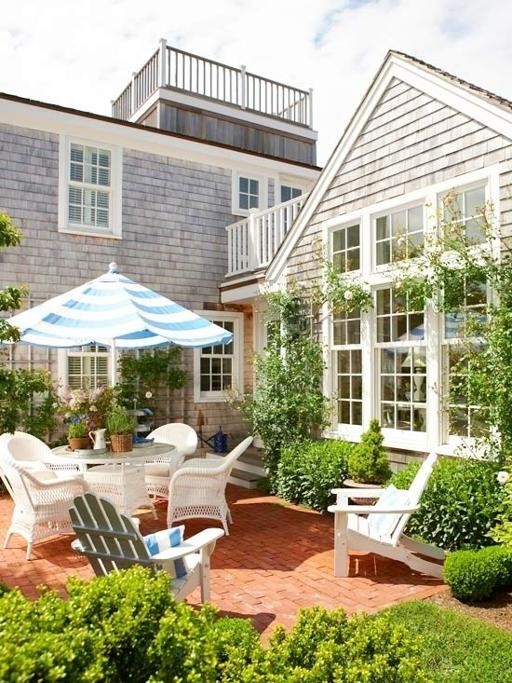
[64,432,109,452]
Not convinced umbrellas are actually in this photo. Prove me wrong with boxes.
[0,262,234,387]
[383,309,489,354]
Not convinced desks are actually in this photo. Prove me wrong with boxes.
[51,444,178,521]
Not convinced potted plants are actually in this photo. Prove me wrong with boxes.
[109,407,138,452]
[341,417,394,507]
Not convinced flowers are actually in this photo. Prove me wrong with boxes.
[52,383,108,431]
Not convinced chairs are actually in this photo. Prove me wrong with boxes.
[145,422,198,500]
[168,435,255,538]
[0,454,89,562]
[0,430,49,459]
[326,452,457,578]
[69,494,226,606]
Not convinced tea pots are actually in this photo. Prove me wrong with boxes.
[89,428,108,450]
